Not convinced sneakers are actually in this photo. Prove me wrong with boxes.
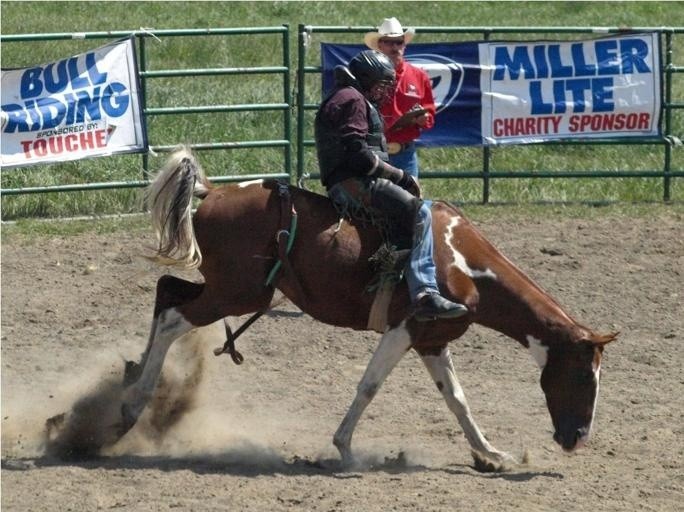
[415,294,467,322]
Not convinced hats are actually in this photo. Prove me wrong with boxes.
[364,17,415,52]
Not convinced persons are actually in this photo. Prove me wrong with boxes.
[312,49,466,320]
[365,17,436,179]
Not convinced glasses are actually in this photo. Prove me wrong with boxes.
[384,40,404,45]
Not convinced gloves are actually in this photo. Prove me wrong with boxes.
[397,169,420,198]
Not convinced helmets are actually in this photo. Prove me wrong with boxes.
[349,50,397,83]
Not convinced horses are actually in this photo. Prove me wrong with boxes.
[117,144,621,470]
